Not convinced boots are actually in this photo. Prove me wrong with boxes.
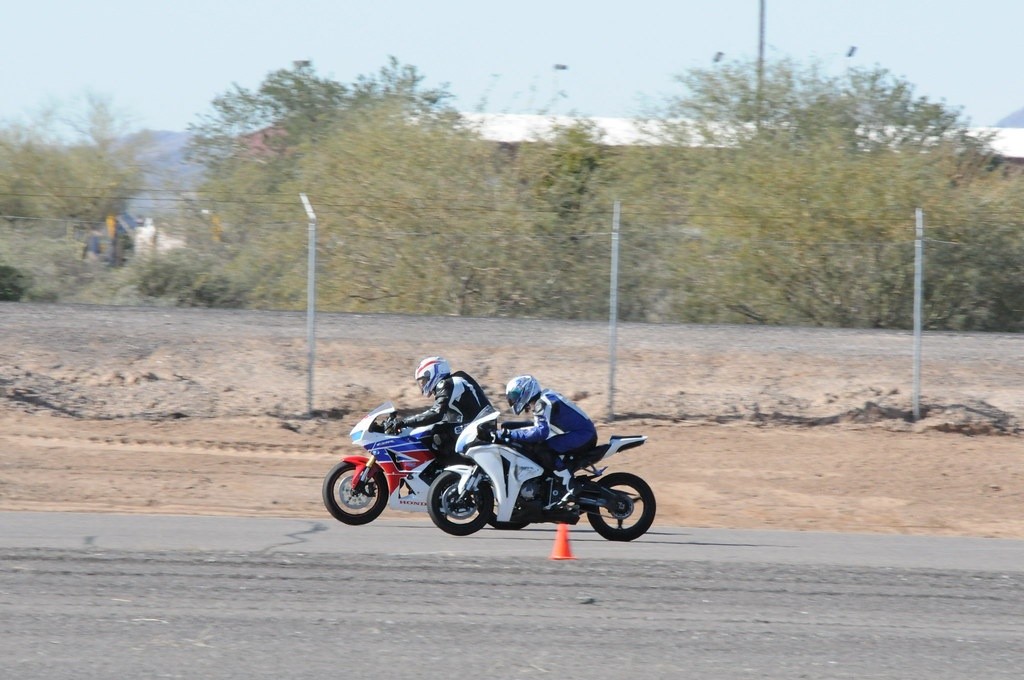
[551,469,582,508]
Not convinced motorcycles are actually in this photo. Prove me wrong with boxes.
[322,401,534,525]
[427,403,657,543]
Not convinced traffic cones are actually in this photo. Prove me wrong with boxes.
[548,524,577,560]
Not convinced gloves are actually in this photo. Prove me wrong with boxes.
[490,428,511,445]
[385,418,407,432]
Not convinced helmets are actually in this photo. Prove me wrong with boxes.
[506,375,542,415]
[415,357,451,398]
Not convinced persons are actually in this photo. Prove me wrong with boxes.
[488,374,598,508]
[384,356,497,469]
